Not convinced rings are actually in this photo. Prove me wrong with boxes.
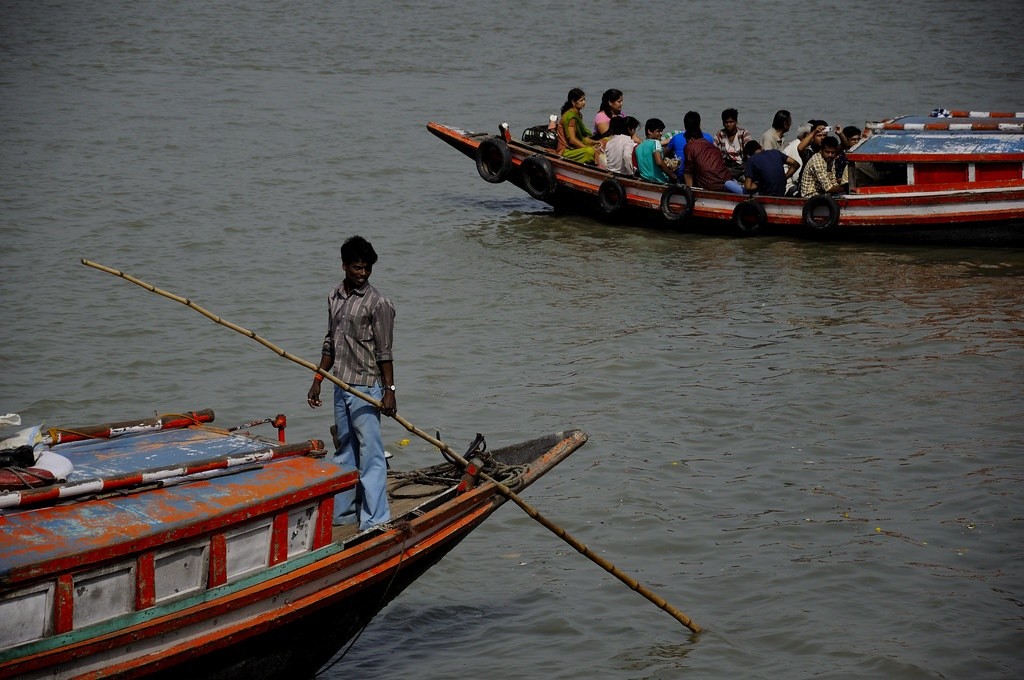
[393,409,394,411]
[308,399,311,400]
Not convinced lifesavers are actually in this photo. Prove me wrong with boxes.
[660,184,696,221]
[476,139,510,182]
[802,195,839,233]
[733,200,765,232]
[519,152,554,199]
[597,178,626,213]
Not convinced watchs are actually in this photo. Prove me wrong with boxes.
[383,385,396,391]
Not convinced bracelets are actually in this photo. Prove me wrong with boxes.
[315,374,324,382]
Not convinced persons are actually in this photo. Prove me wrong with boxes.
[724,140,800,197]
[596,116,640,179]
[781,119,863,198]
[593,88,643,143]
[801,136,849,199]
[683,126,732,192]
[556,88,604,164]
[308,237,397,532]
[713,108,752,183]
[634,118,679,183]
[662,110,714,184]
[754,110,792,152]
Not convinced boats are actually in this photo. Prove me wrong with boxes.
[0,408,592,680]
[425,107,1024,241]
